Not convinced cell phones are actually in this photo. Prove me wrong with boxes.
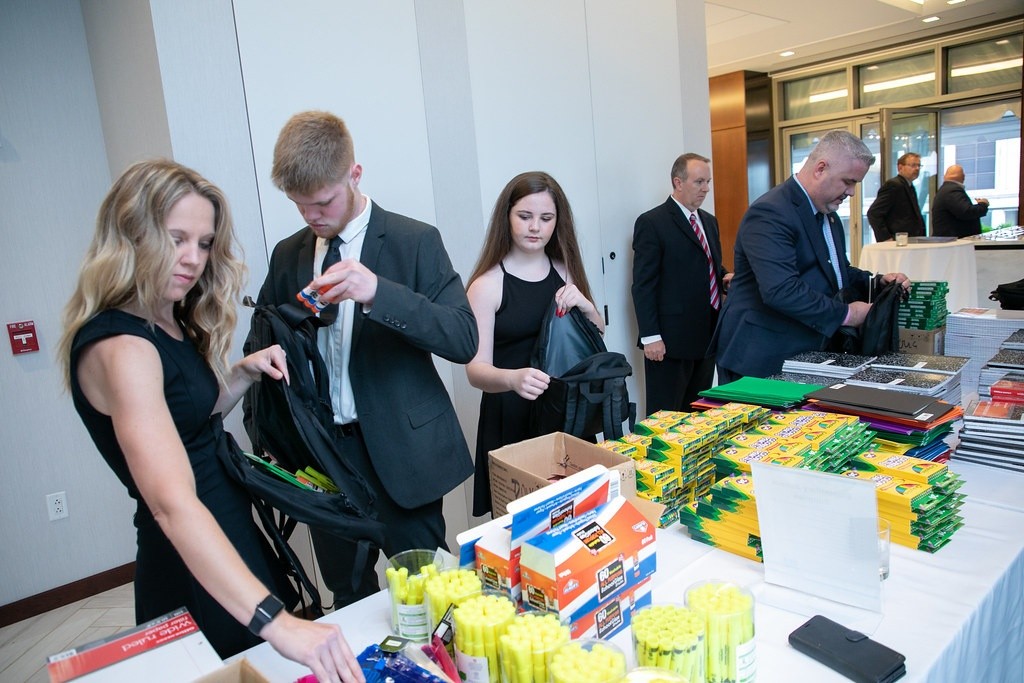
[789,615,906,683]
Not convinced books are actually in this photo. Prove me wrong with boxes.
[782,329,1024,473]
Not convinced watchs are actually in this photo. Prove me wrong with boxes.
[246,595,285,638]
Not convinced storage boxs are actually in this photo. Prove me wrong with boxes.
[898,326,945,357]
[430,401,969,653]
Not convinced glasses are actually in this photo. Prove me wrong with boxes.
[905,163,923,168]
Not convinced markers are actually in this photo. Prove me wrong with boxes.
[386,563,482,656]
[295,466,339,493]
[498,610,695,683]
[453,590,517,683]
[630,580,762,683]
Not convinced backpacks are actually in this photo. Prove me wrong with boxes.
[989,279,1024,310]
[243,296,334,466]
[530,295,637,441]
[210,365,386,613]
[831,274,909,357]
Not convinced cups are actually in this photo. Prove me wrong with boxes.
[877,520,889,581]
[384,549,755,683]
[895,232,908,246]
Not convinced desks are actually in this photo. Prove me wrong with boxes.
[223,388,1024,683]
[864,238,976,314]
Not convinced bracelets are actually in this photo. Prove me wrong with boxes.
[236,360,261,382]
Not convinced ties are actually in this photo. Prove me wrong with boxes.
[690,214,720,312]
[822,214,843,290]
[277,235,344,330]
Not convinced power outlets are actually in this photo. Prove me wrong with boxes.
[46,491,69,521]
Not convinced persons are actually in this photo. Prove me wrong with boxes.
[57,159,365,683]
[631,152,734,420]
[465,172,604,520]
[867,152,926,243]
[931,164,989,239]
[242,110,480,613]
[707,130,911,387]
